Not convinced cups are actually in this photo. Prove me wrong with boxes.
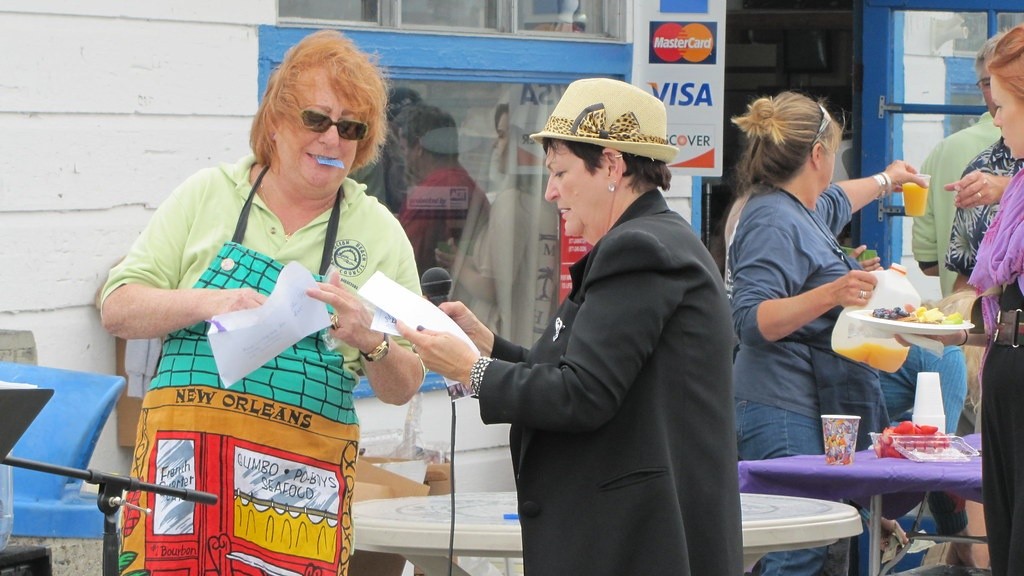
[913,371,946,436]
[821,415,860,465]
[902,174,932,216]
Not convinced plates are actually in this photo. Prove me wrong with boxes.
[848,310,976,334]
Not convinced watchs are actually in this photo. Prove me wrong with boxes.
[358,333,390,362]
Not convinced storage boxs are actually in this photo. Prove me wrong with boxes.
[358,452,461,576]
[342,457,431,576]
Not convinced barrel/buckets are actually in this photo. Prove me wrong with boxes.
[830,262,923,372]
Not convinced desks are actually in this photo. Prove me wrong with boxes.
[352,485,865,576]
[733,429,1007,576]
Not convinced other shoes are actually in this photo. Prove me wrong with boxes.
[944,544,976,568]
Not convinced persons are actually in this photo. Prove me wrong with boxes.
[910,110,1002,298]
[730,89,929,576]
[396,76,746,576]
[945,29,1024,294]
[877,290,987,576]
[92,30,424,576]
[966,25,1024,576]
[348,85,535,338]
[721,145,754,301]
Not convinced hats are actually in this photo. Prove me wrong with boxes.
[528,77,681,165]
[387,87,422,121]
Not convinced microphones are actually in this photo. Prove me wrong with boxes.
[419,265,467,402]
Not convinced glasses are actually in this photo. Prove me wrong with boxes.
[976,77,992,92]
[277,102,370,141]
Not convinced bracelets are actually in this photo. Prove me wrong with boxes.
[870,172,892,200]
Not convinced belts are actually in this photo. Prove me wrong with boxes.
[992,310,1024,349]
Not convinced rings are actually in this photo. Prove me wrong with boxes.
[330,314,339,331]
[859,290,866,299]
[977,191,982,198]
[412,344,418,354]
